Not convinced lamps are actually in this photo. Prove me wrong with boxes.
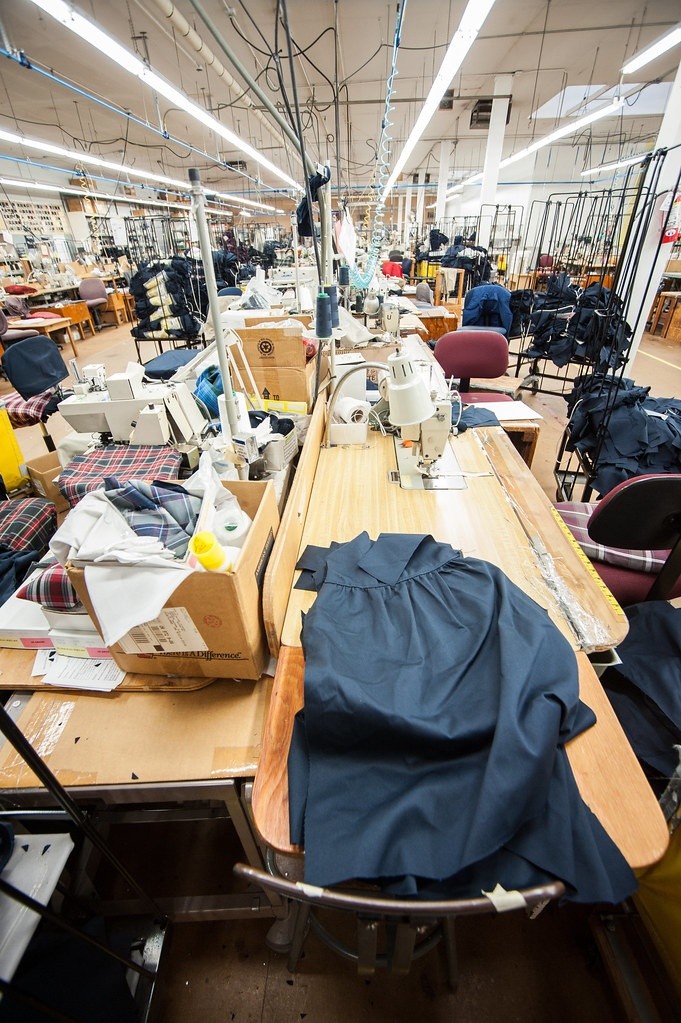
[0,0,681,214]
[363,287,380,315]
[324,347,437,449]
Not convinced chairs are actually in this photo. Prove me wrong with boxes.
[5,296,65,350]
[146,348,203,379]
[218,287,243,296]
[539,255,553,272]
[458,284,512,377]
[436,330,515,404]
[402,257,412,275]
[79,278,118,333]
[1,335,74,453]
[390,255,403,262]
[382,261,402,279]
[552,473,681,607]
[0,308,40,382]
[390,250,400,258]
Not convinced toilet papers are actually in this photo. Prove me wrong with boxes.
[333,396,371,424]
[213,509,252,546]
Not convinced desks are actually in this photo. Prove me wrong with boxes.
[0,259,681,993]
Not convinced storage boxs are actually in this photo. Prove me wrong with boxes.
[326,351,367,402]
[130,209,145,215]
[86,264,102,273]
[124,186,136,196]
[227,315,324,413]
[80,265,86,274]
[63,478,280,682]
[160,192,177,201]
[326,328,402,390]
[264,425,300,470]
[0,277,11,287]
[26,450,70,512]
[103,264,114,272]
[65,196,96,214]
[105,373,143,401]
[70,178,98,190]
[325,394,370,444]
[33,273,48,286]
[232,432,260,463]
[57,261,81,275]
